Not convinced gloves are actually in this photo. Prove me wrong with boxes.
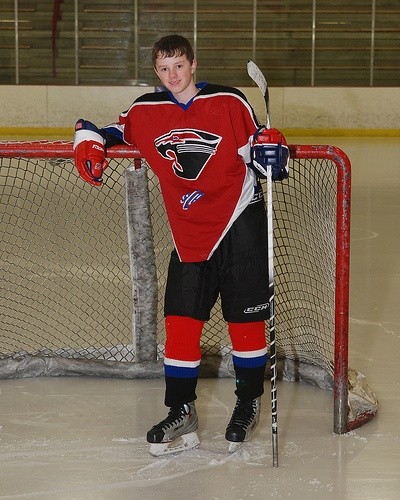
[74,119,107,186]
[252,125,289,181]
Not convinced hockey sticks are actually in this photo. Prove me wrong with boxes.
[246,60,279,468]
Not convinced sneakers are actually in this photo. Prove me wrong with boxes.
[147,401,200,456]
[225,397,261,452]
